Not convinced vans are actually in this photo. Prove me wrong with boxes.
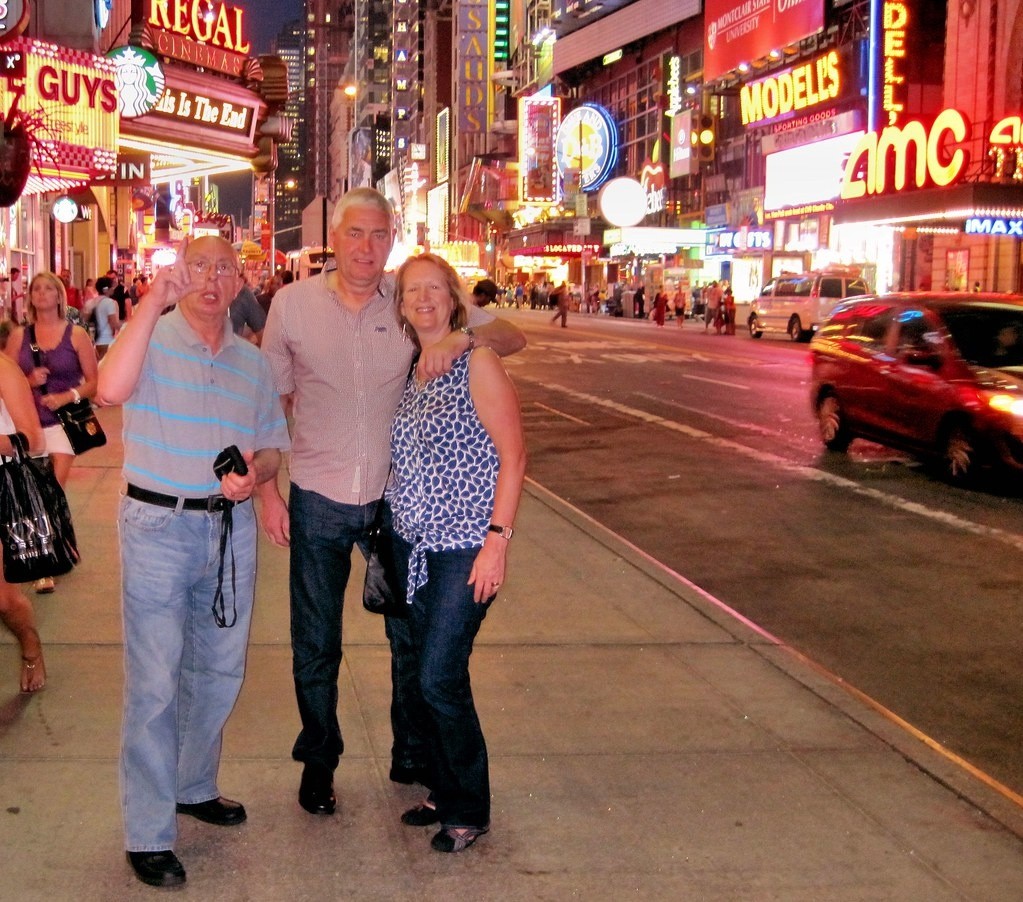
[746,273,872,344]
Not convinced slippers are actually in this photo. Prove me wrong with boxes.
[21,627,48,693]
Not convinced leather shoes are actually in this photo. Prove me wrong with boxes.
[174,791,246,828]
[125,835,186,886]
[298,759,337,815]
[390,753,440,790]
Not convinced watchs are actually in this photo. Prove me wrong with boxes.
[70,389,81,404]
[489,525,513,539]
[461,327,475,350]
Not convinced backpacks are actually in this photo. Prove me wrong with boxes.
[86,296,112,343]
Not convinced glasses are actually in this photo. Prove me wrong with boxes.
[184,259,241,277]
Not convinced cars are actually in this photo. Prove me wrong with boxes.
[806,288,1023,492]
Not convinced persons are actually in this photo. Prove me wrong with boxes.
[674,287,686,327]
[495,280,555,309]
[384,252,525,852]
[916,284,925,291]
[99,236,292,886]
[0,268,131,360]
[655,285,668,327]
[993,324,1018,366]
[469,280,496,309]
[230,271,294,345]
[636,285,645,317]
[552,281,567,328]
[973,282,980,291]
[256,186,528,818]
[591,285,598,314]
[692,280,735,336]
[0,350,46,692]
[6,272,98,592]
[130,273,153,313]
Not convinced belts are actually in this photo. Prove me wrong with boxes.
[126,482,249,513]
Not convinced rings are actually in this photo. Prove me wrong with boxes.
[491,583,499,586]
[169,267,176,272]
[41,375,44,378]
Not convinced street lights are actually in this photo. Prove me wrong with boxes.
[319,84,357,269]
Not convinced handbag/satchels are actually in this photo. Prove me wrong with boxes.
[0,434,81,584]
[362,527,410,621]
[57,398,107,454]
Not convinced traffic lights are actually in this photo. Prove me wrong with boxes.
[697,112,715,162]
[688,110,701,165]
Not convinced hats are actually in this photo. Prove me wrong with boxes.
[478,278,500,305]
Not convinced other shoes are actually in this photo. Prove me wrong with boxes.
[401,797,447,826]
[432,823,491,853]
